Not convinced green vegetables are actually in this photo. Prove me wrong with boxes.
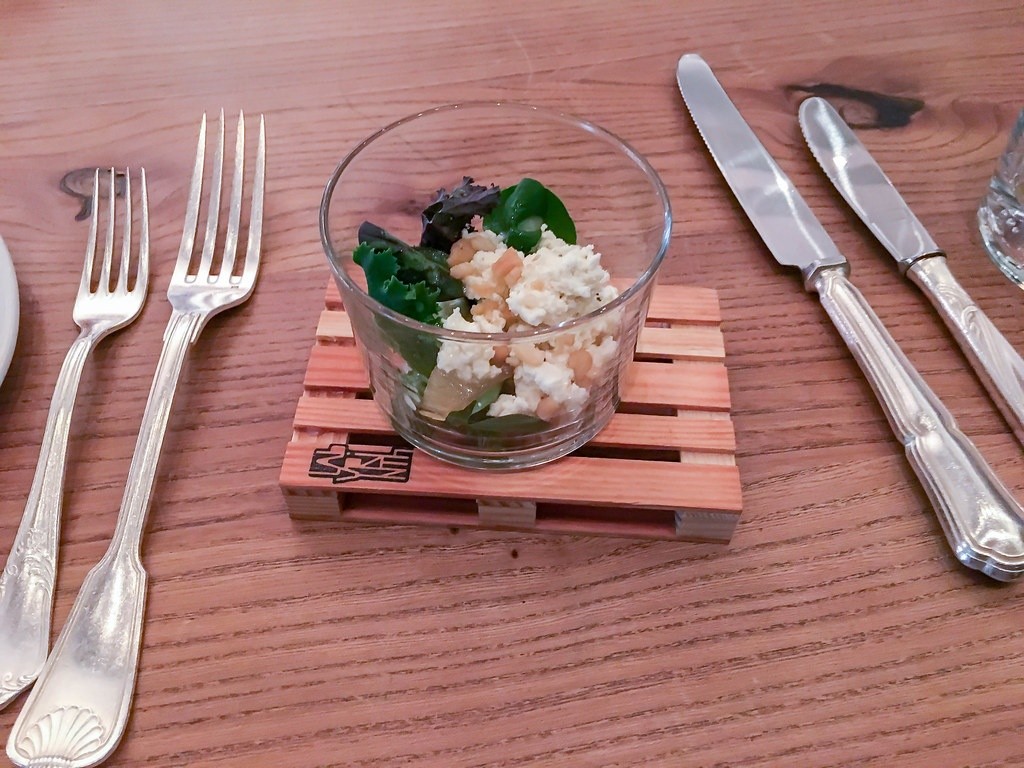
[350,178,577,435]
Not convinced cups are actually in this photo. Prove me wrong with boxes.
[320,100,672,472]
[976,106,1024,290]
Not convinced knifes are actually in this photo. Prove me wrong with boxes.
[676,54,1024,585]
[799,97,1023,451]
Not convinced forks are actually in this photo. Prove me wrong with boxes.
[6,109,264,767]
[0,168,149,708]
